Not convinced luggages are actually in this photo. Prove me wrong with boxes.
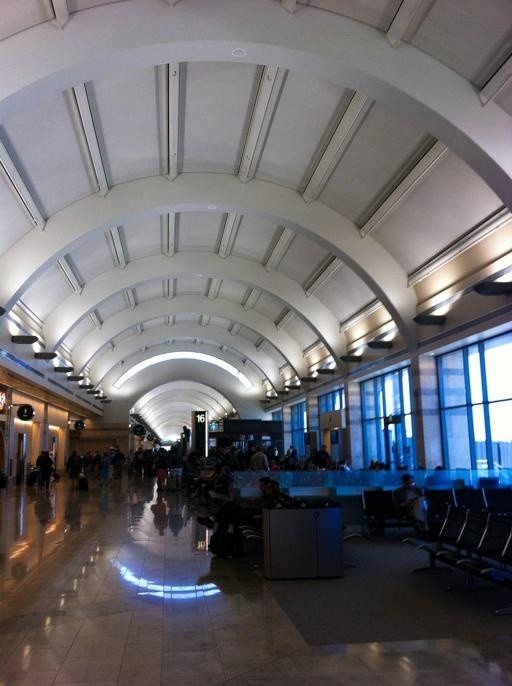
[79,472,88,490]
[167,466,177,490]
[26,469,40,486]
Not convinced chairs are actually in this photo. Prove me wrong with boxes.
[364,486,511,617]
[234,492,362,569]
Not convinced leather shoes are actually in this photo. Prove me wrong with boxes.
[196,516,214,529]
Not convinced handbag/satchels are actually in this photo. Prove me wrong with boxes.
[209,531,246,562]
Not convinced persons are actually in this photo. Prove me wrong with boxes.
[36,425,194,492]
[196,555,263,607]
[195,442,446,572]
[34,474,195,538]
